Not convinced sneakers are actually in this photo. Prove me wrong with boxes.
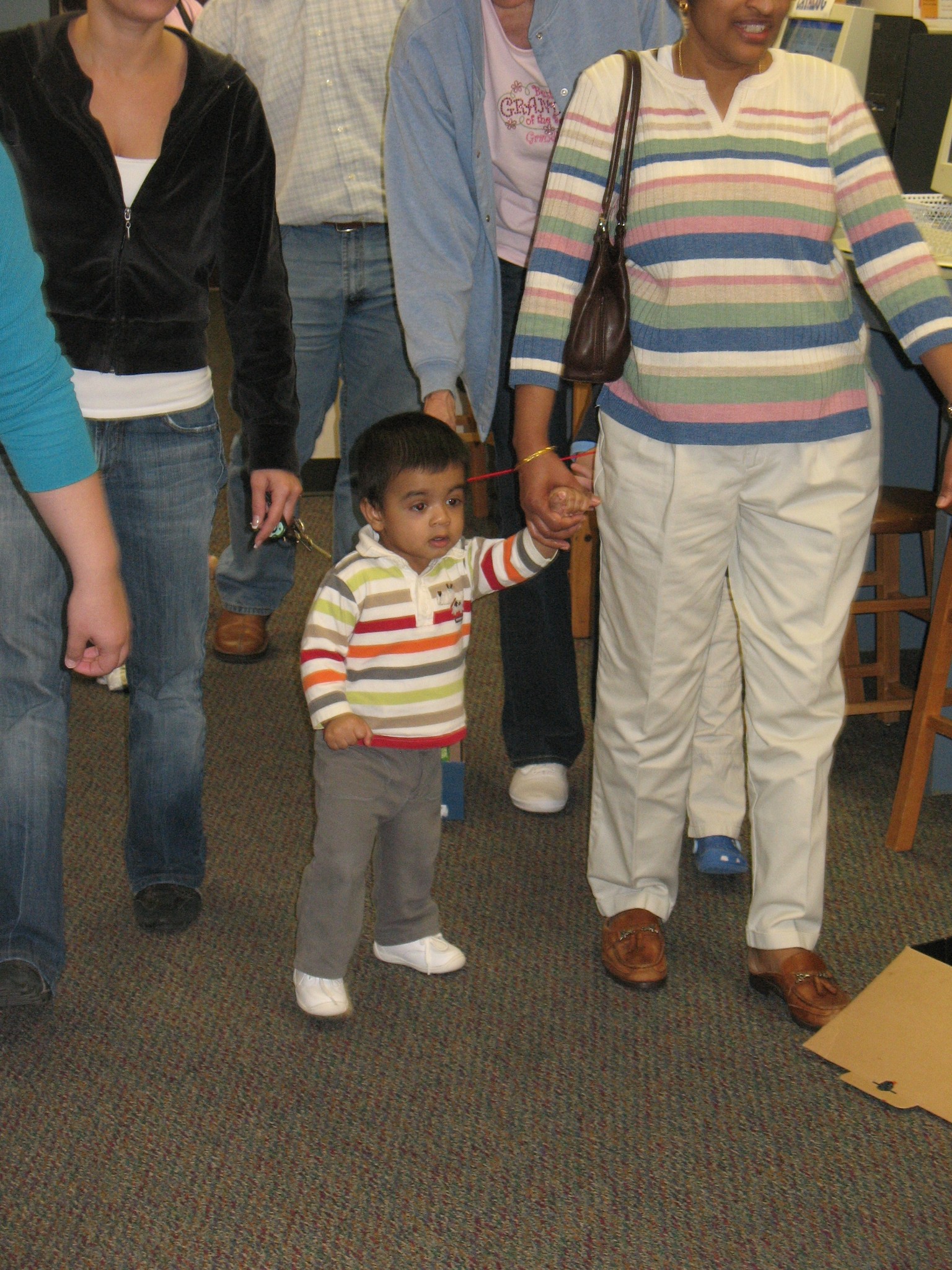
[294,969,350,1017]
[134,882,202,932]
[0,960,51,1006]
[372,930,467,975]
[508,762,568,812]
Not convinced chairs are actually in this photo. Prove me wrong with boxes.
[455,381,943,719]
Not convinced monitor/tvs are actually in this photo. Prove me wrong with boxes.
[772,3,874,98]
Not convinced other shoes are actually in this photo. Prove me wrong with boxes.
[747,950,850,1030]
[214,609,267,664]
[602,908,669,989]
[95,664,129,690]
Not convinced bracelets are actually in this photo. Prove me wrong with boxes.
[512,444,557,472]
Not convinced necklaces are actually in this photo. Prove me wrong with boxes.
[679,34,765,79]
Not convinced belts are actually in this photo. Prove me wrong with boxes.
[326,222,381,232]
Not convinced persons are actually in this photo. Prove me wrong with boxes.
[292,412,601,1020]
[190,0,422,658]
[0,0,303,1005]
[510,0,952,1029]
[384,0,692,812]
[0,141,130,677]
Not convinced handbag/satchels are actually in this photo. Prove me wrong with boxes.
[558,47,640,381]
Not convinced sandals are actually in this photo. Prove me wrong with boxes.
[691,835,747,875]
